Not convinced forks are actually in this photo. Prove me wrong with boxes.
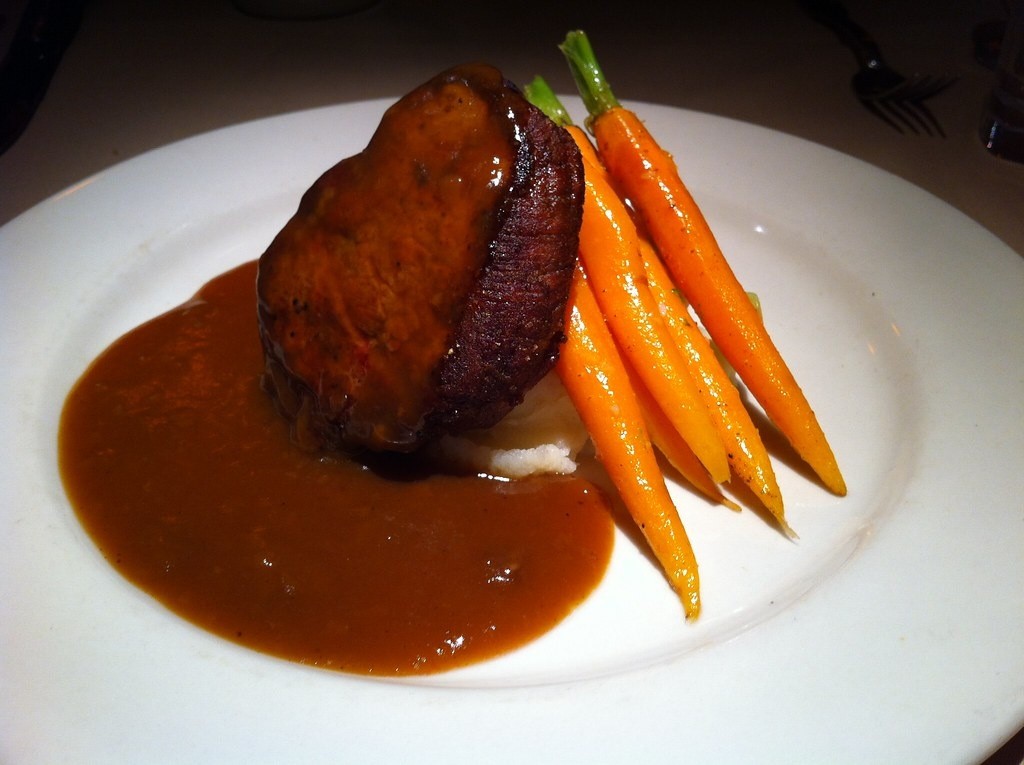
[802,0,969,103]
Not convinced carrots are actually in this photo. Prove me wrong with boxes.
[546,107,848,621]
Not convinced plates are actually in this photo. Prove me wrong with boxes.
[1,84,1024,764]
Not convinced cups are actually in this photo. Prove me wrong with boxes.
[980,0,1024,165]
